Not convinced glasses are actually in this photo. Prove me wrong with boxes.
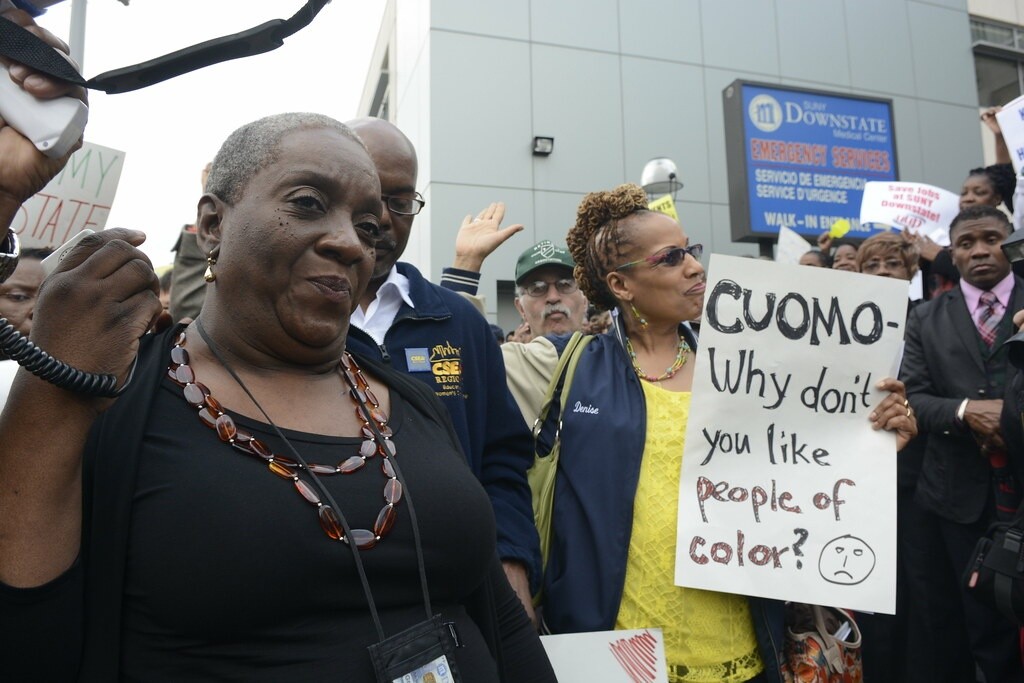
[864,259,904,273]
[613,244,702,272]
[521,278,577,297]
[382,192,424,216]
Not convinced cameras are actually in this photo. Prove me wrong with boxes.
[1000,230,1024,369]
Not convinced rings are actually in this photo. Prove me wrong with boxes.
[907,408,911,418]
[474,218,481,221]
[904,398,908,408]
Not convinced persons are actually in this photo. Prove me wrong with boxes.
[0,0,1023,683]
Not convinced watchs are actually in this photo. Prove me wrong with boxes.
[0,227,20,284]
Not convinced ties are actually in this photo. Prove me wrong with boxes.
[977,292,1001,350]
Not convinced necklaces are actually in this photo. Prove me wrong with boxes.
[625,334,690,380]
[169,331,404,549]
[165,350,377,474]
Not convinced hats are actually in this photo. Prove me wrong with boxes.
[515,240,576,283]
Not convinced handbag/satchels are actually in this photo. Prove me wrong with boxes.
[526,331,594,609]
[963,520,1024,626]
[779,602,863,683]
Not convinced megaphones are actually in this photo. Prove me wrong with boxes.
[0,0,131,157]
